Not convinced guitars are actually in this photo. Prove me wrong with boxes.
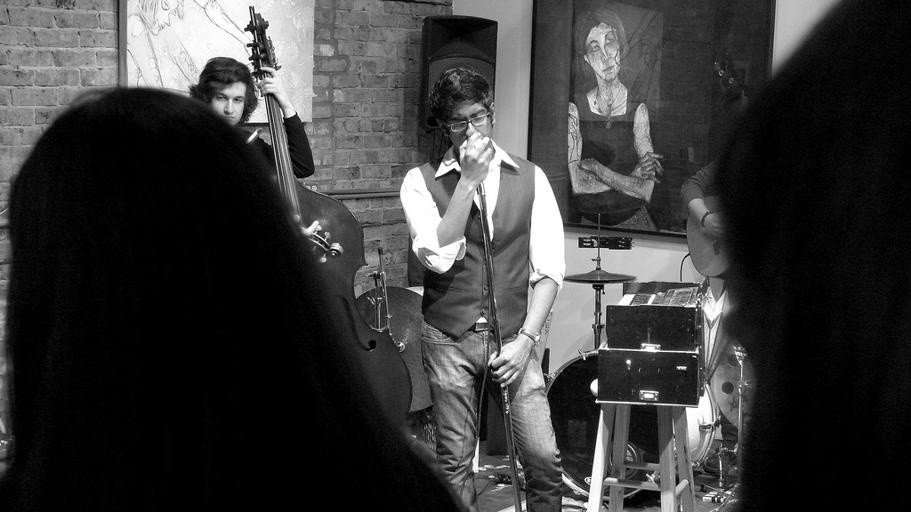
[687,197,742,280]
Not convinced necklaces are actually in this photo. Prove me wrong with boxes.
[597,81,624,133]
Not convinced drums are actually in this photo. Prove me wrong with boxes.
[545,340,719,499]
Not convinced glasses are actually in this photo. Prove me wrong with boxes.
[438,112,490,132]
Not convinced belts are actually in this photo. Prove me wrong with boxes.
[468,322,496,333]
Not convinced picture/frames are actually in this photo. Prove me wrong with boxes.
[527,0,777,244]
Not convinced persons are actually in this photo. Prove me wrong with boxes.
[189,56,316,179]
[399,65,567,512]
[680,155,739,470]
[2,86,465,511]
[717,1,911,510]
[568,5,667,229]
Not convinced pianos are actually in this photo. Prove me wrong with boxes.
[607,281,710,350]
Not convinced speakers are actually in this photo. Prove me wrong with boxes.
[418,14,498,151]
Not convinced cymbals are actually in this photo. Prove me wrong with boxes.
[355,285,434,413]
[562,269,636,284]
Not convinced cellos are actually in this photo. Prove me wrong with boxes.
[245,7,413,432]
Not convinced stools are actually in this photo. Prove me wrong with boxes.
[587,377,695,511]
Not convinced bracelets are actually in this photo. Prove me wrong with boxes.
[700,210,713,227]
[518,328,542,344]
